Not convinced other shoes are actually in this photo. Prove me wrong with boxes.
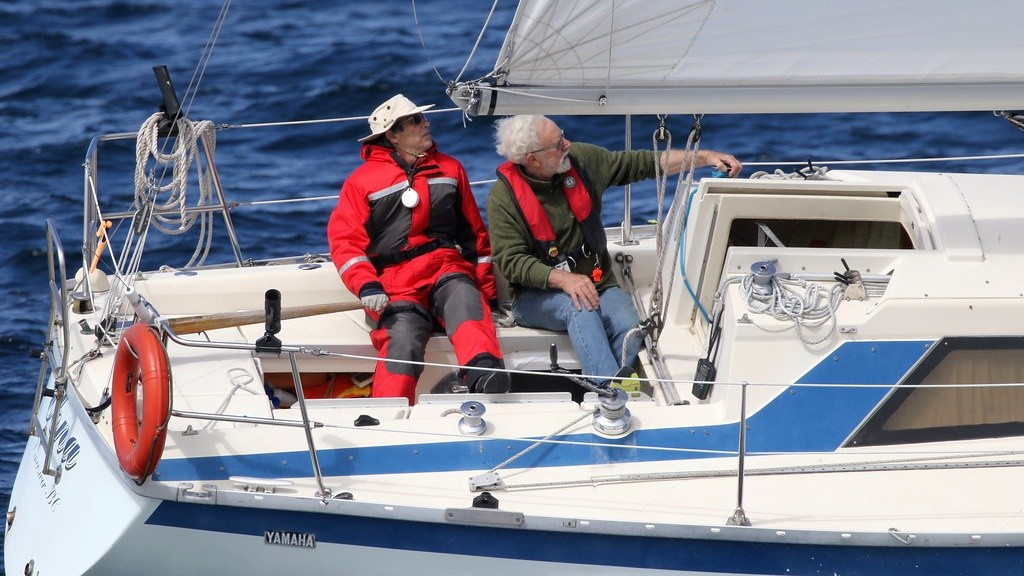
[610,367,641,391]
[476,370,511,393]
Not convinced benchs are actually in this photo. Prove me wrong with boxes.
[240,302,584,377]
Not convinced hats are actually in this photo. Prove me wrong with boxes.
[358,94,436,143]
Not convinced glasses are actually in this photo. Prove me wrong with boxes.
[527,129,564,155]
[399,114,427,125]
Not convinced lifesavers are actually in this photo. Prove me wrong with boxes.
[111,322,170,482]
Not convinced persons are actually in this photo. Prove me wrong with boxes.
[326,94,510,406]
[487,112,743,385]
